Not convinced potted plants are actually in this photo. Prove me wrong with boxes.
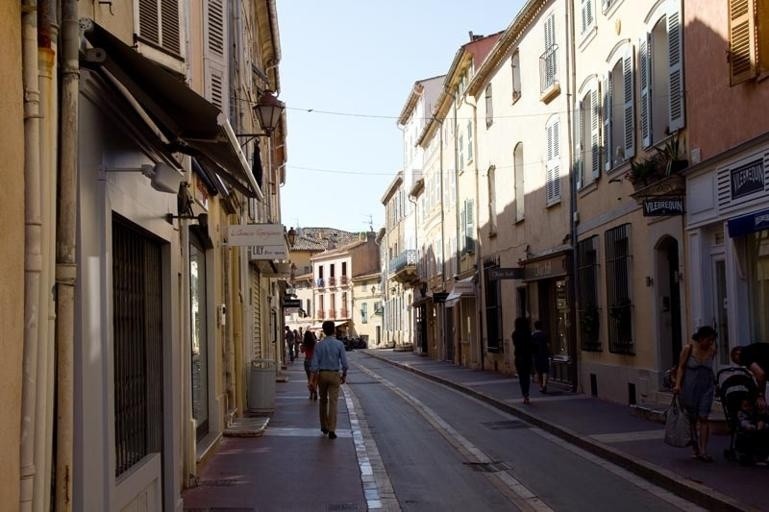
[625,134,690,192]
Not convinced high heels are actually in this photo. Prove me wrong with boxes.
[522,396,530,405]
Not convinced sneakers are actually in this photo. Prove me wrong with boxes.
[538,386,548,395]
[320,427,338,440]
[309,391,318,401]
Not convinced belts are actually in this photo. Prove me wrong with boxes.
[320,369,339,372]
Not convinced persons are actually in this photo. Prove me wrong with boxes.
[737,399,765,431]
[308,321,349,439]
[672,326,717,461]
[512,317,533,405]
[731,342,769,408]
[531,321,549,394]
[285,326,317,401]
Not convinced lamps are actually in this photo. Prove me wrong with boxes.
[105,161,182,194]
[236,88,286,147]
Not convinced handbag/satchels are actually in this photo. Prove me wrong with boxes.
[662,390,697,448]
[663,365,678,387]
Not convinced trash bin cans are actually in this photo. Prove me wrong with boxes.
[247,359,277,417]
